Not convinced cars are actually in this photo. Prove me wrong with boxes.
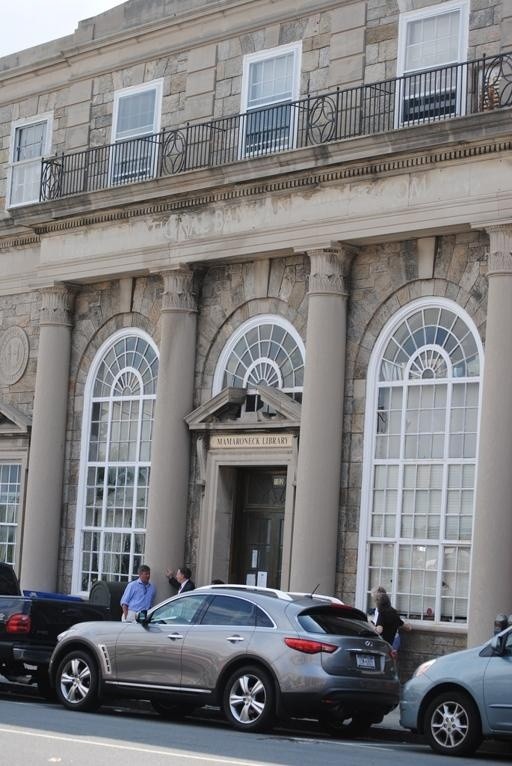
[397,624,512,755]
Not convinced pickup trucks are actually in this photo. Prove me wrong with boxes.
[0,559,112,697]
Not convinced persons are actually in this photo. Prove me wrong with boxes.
[375,594,412,681]
[370,587,388,626]
[119,564,157,623]
[164,567,195,596]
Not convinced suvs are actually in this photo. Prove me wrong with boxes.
[48,586,401,735]
[192,583,344,607]
